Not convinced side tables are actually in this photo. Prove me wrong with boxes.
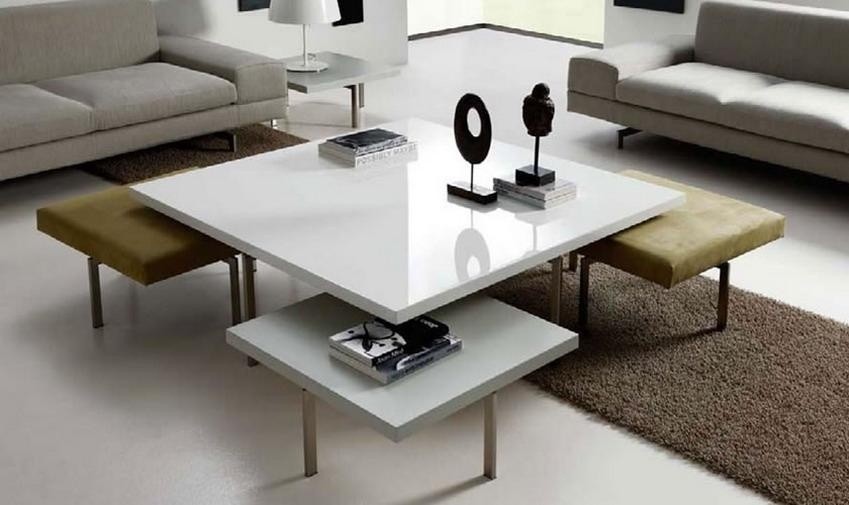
[275,52,402,129]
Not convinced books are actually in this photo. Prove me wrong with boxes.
[494,184,578,209]
[329,314,449,368]
[327,333,463,385]
[318,136,419,168]
[493,171,576,200]
[327,128,407,156]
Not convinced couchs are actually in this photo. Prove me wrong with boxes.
[566,0,848,184]
[0,0,290,186]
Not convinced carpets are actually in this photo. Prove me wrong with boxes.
[80,125,849,503]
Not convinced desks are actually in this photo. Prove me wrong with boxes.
[125,120,690,370]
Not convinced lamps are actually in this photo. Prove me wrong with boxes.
[268,0,341,73]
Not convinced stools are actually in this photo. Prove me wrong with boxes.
[36,172,245,340]
[225,291,579,485]
[558,168,787,334]
